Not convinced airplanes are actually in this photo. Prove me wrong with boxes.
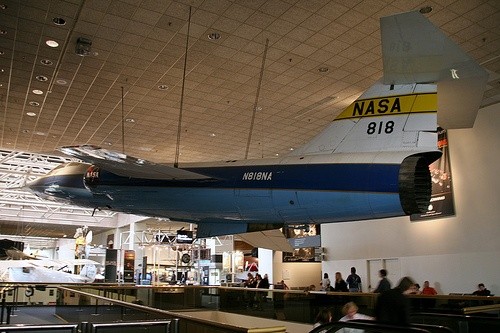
[0,246,102,284]
[27,8,492,254]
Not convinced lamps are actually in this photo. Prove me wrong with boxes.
[75,38,91,56]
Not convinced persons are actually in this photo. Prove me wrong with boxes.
[345,267,362,293]
[374,277,414,333]
[470,283,492,296]
[334,272,349,292]
[313,310,333,333]
[245,272,268,309]
[420,280,438,295]
[405,283,421,295]
[320,273,330,291]
[334,301,375,333]
[371,269,391,293]
[170,271,187,285]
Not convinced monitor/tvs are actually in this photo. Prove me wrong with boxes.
[177,230,193,243]
